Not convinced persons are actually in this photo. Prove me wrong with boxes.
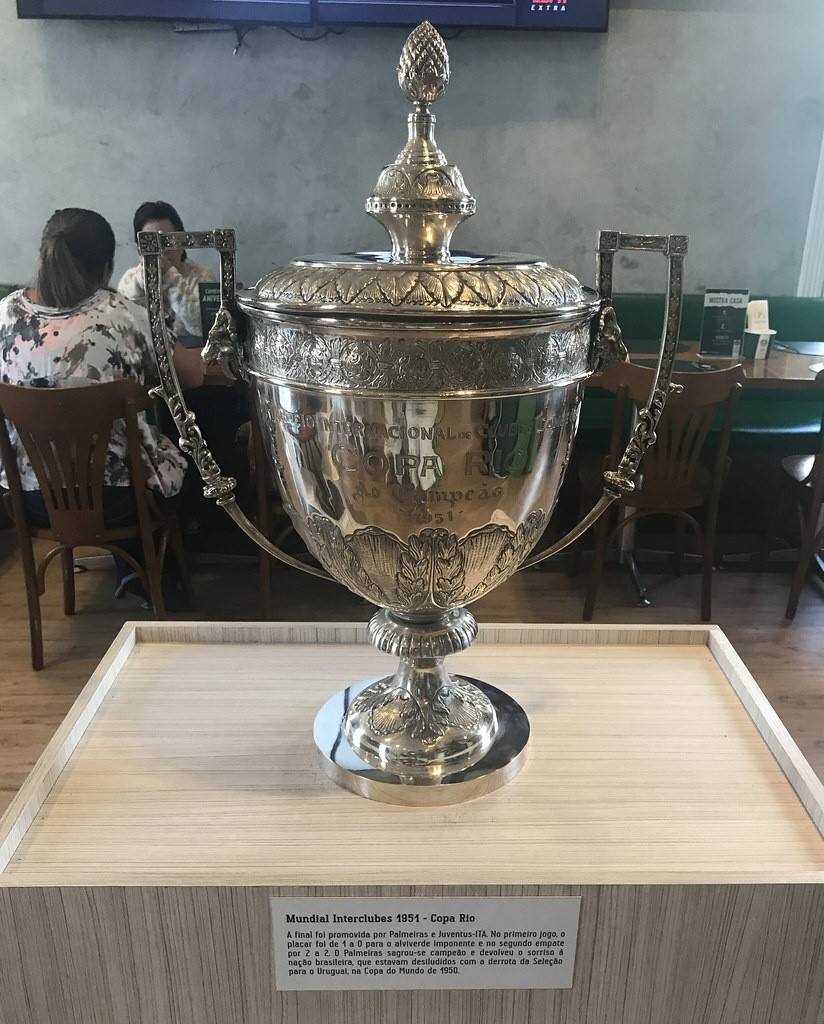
[114,200,219,531]
[0,207,208,605]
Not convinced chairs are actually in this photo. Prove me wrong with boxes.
[576,355,746,623]
[0,378,182,671]
[777,369,824,620]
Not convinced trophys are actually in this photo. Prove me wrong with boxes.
[135,17,691,805]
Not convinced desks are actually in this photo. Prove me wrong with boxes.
[536,340,824,609]
[73,346,276,607]
